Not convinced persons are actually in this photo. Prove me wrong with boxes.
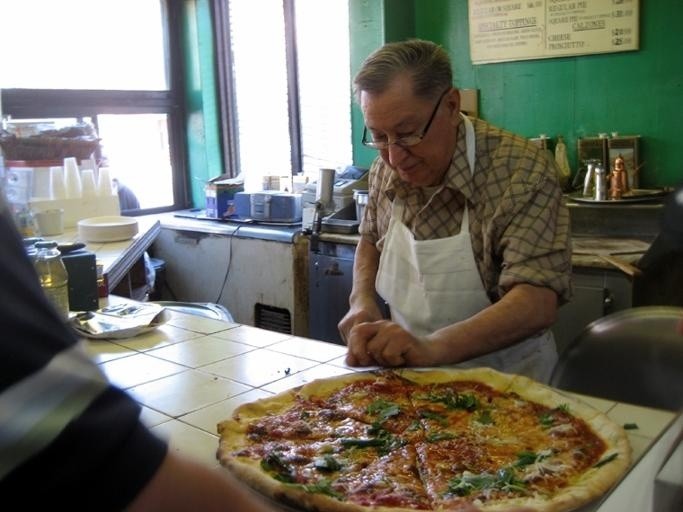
[0,179,271,512]
[337,39,573,387]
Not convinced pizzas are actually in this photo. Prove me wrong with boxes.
[215,364,631,511]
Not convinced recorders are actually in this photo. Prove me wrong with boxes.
[234,192,303,222]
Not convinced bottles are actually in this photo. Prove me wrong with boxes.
[591,160,606,200]
[554,135,570,179]
[21,236,44,264]
[32,241,70,325]
[18,202,36,237]
[581,163,594,196]
[608,155,628,199]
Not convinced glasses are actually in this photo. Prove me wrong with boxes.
[361,84,453,150]
